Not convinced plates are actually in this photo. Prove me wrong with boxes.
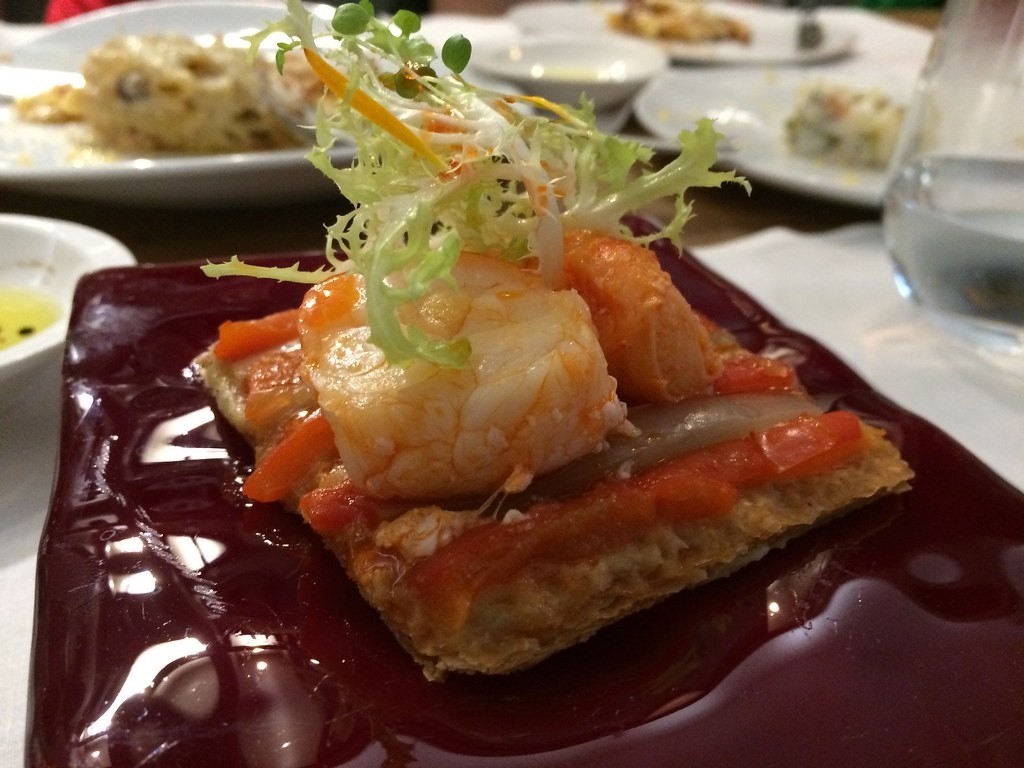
[0,1,530,203]
[387,14,636,137]
[25,212,1023,767]
[631,59,913,209]
[506,1,853,64]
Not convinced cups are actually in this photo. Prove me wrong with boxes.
[880,0,1024,349]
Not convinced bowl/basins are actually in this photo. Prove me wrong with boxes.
[477,33,658,117]
[0,214,138,384]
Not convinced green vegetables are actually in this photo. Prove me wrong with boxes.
[199,1,753,372]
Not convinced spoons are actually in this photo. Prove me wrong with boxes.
[799,0,824,51]
